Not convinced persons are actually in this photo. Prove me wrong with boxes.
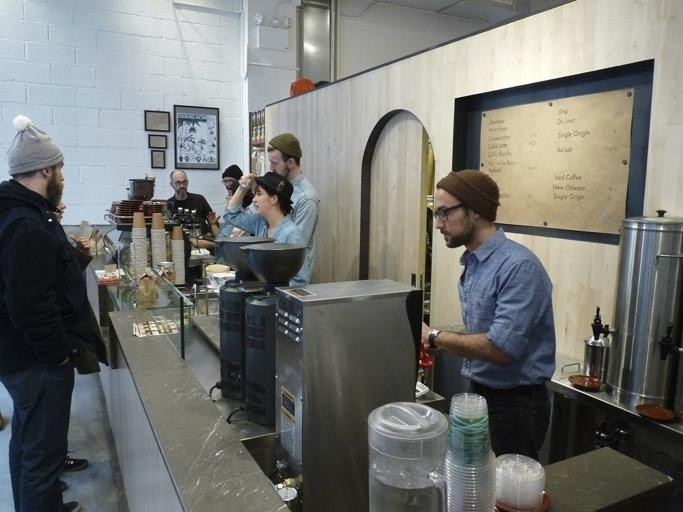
[422,168,556,464]
[0,113,110,512]
[55,202,96,472]
[166,168,220,238]
[207,131,320,286]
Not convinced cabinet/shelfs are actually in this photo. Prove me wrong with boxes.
[248,110,265,177]
[548,391,683,511]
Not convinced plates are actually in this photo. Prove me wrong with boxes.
[496,493,551,512]
[112,199,165,222]
[636,404,675,422]
[568,374,601,391]
[189,249,210,259]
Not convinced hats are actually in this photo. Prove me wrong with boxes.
[437,169,501,220]
[269,133,302,158]
[255,171,295,203]
[222,165,243,180]
[7,115,64,175]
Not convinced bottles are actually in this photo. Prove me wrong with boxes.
[583,335,610,383]
[172,207,202,237]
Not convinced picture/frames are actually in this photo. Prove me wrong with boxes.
[143,109,170,132]
[151,150,165,168]
[148,134,167,149]
[174,105,220,170]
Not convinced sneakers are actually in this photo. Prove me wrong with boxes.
[62,500,81,512]
[60,479,67,492]
[63,459,88,472]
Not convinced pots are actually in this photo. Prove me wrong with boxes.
[126,179,155,201]
[604,209,683,410]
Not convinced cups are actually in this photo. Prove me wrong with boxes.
[132,211,185,288]
[444,391,496,512]
[205,264,238,295]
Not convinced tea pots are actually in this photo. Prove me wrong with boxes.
[367,400,447,512]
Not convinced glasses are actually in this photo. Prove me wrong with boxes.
[431,203,465,221]
[172,180,189,185]
[221,179,235,185]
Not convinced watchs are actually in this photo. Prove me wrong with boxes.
[427,327,441,349]
